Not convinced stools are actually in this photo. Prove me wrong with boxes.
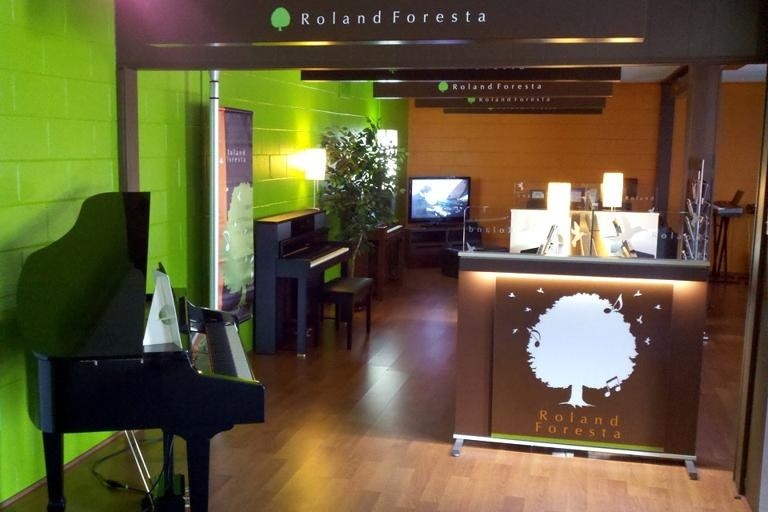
[319,277,372,351]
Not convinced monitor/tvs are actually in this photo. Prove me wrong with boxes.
[408,176,471,228]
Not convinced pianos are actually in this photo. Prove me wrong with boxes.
[20,190,265,512]
[254,204,352,356]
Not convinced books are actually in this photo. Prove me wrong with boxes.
[699,239,708,253]
[683,215,696,242]
[687,169,700,180]
[681,250,686,260]
[701,181,709,204]
[700,202,710,223]
[685,197,694,218]
[700,217,710,236]
[682,232,695,260]
[687,178,697,200]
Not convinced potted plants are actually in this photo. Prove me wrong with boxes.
[317,117,409,312]
